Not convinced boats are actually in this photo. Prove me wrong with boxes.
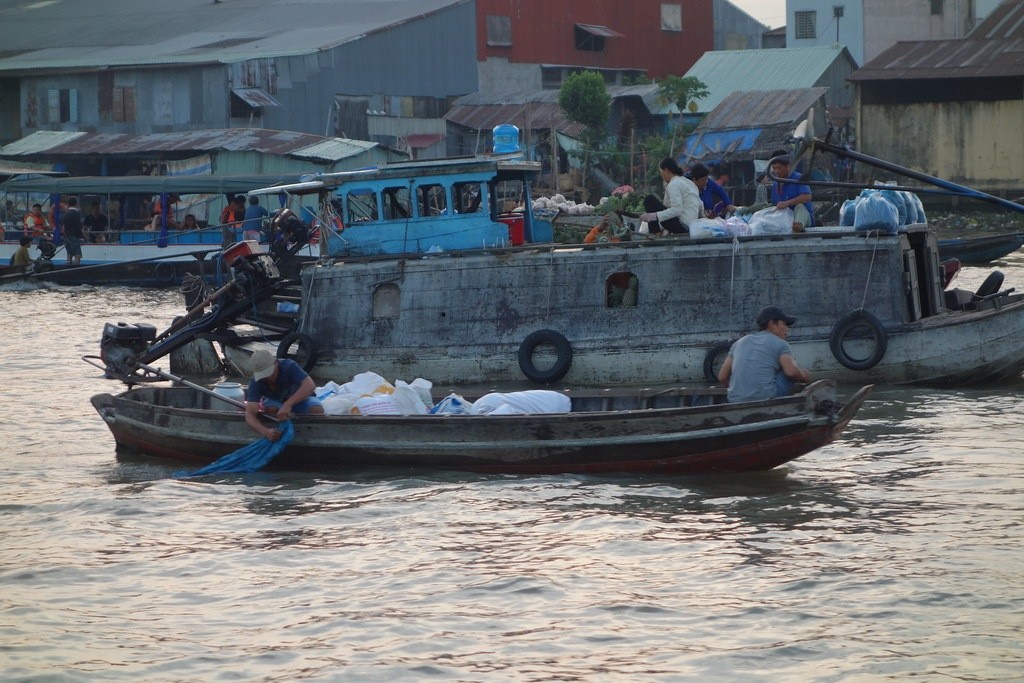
[79,352,875,478]
[178,154,1024,391]
[222,148,555,335]
[0,153,337,287]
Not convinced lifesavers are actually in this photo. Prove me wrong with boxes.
[276,332,318,374]
[975,270,1005,297]
[704,338,738,382]
[516,330,573,382]
[829,310,888,370]
[154,260,176,284]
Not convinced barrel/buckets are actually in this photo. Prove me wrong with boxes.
[210,382,244,410]
[497,213,524,245]
[492,123,520,152]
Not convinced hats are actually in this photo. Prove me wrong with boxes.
[250,350,277,381]
[756,305,798,327]
[171,192,183,203]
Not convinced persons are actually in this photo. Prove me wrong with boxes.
[640,158,699,237]
[718,306,811,402]
[183,215,196,229]
[61,197,83,264]
[755,174,768,204]
[242,196,276,242]
[23,203,51,238]
[83,202,108,243]
[222,195,246,228]
[14,236,33,266]
[144,213,161,231]
[770,150,816,232]
[685,163,736,212]
[245,349,324,440]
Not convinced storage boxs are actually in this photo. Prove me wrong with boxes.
[224,238,281,295]
